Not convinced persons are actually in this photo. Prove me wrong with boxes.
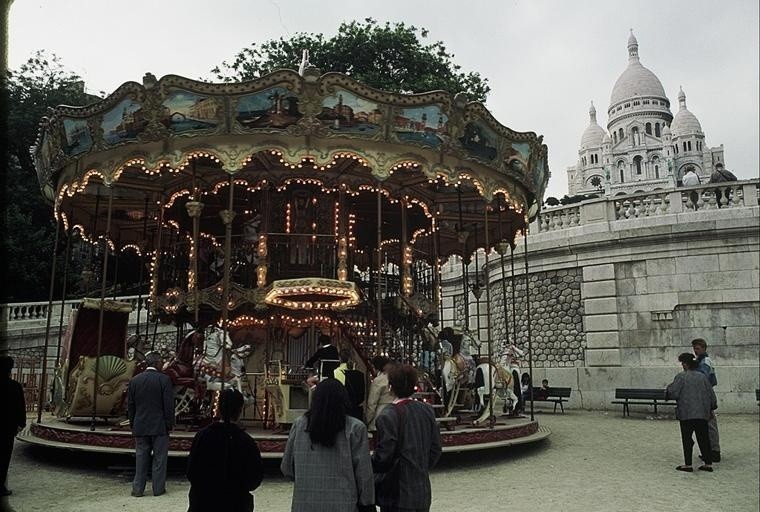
[0,356,26,512]
[710,162,737,209]
[682,166,701,211]
[664,353,717,472]
[279,334,442,511]
[127,352,174,497]
[533,379,550,400]
[692,338,721,462]
[520,372,530,412]
[183,389,264,512]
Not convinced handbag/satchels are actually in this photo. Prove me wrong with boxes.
[369,456,398,507]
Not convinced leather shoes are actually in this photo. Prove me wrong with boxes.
[1,488,12,496]
[676,452,721,472]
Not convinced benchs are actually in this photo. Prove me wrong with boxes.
[612,387,678,417]
[526,385,571,414]
